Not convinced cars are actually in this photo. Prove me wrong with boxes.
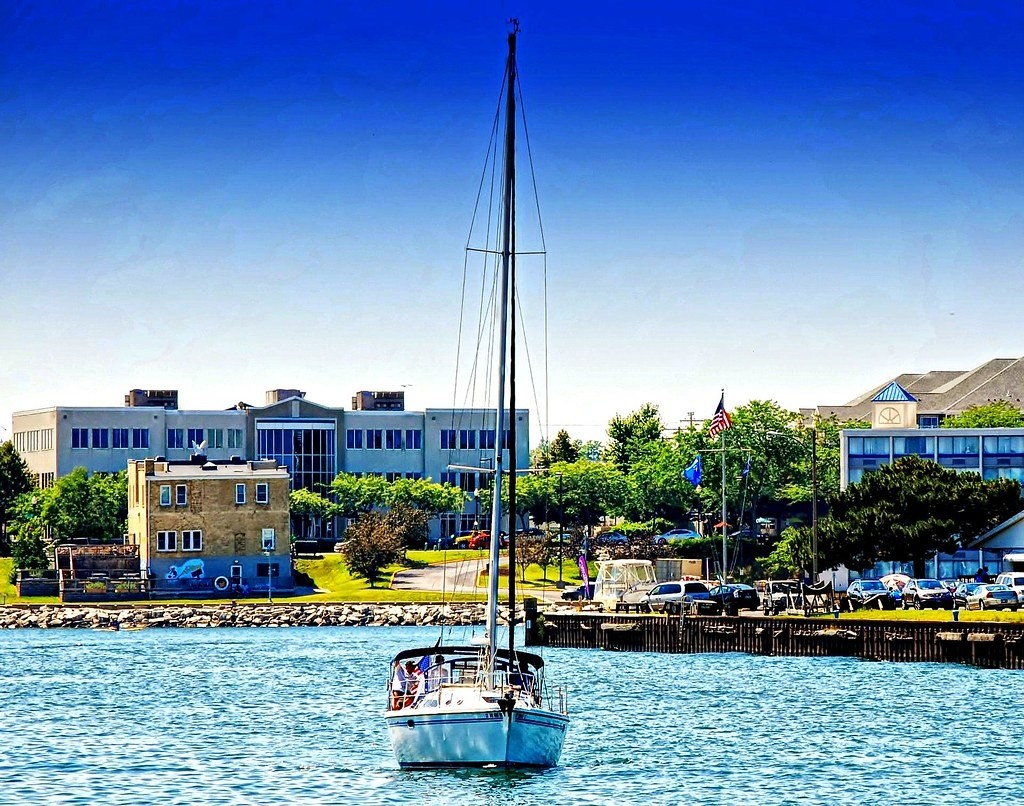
[425,530,546,550]
[596,531,629,546]
[727,529,752,540]
[952,583,989,610]
[652,528,702,546]
[708,584,760,612]
[965,584,1019,612]
[550,533,575,547]
[941,579,962,594]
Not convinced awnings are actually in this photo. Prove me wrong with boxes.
[1003,553,1024,562]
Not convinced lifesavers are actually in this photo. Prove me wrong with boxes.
[214,575,229,591]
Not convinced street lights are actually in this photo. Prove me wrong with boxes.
[765,429,818,585]
[555,471,563,582]
[696,484,702,522]
[473,487,480,530]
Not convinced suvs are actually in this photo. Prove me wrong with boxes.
[845,578,894,613]
[637,581,711,615]
[763,580,803,616]
[900,578,954,611]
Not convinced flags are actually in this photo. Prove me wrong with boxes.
[410,655,430,694]
[742,460,751,477]
[684,454,701,486]
[710,398,731,437]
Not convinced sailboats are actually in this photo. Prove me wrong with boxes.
[382,14,571,773]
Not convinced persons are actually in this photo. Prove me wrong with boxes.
[975,567,988,583]
[432,655,448,683]
[394,660,416,696]
[234,582,249,598]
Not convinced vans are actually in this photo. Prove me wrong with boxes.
[996,571,1024,608]
